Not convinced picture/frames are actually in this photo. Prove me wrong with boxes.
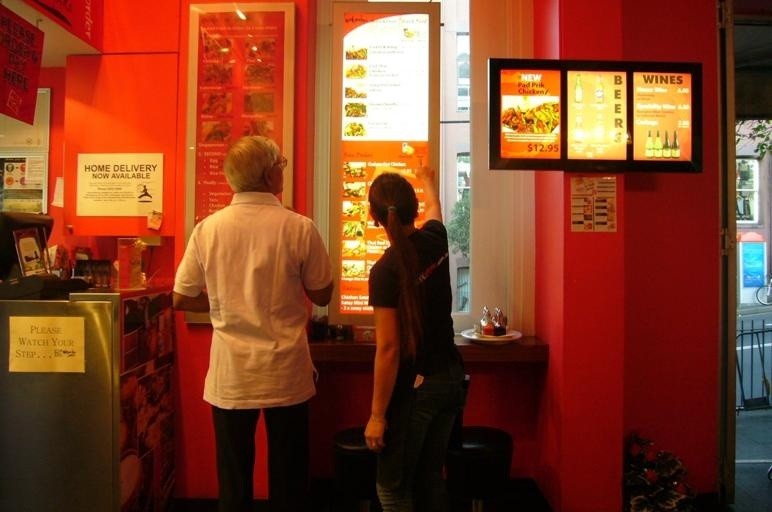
[184,0,441,327]
[486,57,703,174]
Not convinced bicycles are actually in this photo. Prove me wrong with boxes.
[755,273,772,306]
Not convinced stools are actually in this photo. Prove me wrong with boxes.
[343,424,514,512]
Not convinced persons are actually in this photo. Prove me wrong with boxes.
[171,133,337,512]
[359,155,468,512]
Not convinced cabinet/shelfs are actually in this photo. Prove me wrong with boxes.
[0,285,178,512]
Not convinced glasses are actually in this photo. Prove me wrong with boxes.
[273,154,287,168]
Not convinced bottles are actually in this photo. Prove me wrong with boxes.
[574,74,585,103]
[671,130,681,159]
[644,129,653,157]
[594,75,605,105]
[662,130,671,158]
[654,130,662,158]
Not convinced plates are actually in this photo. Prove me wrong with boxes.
[460,327,523,343]
